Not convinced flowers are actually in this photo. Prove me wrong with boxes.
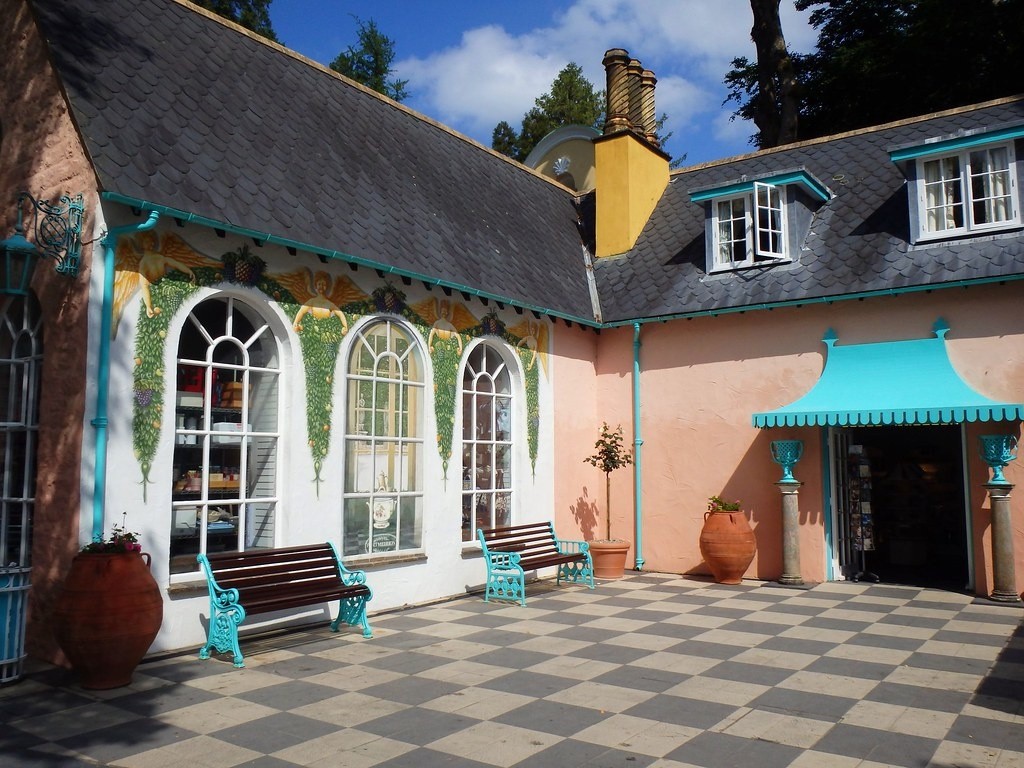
[76,511,143,553]
[707,495,744,511]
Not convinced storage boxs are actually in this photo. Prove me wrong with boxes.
[173,510,197,528]
[176,391,204,407]
[219,381,254,408]
[212,422,253,444]
[210,480,239,488]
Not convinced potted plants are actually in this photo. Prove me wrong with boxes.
[581,421,634,579]
[699,511,757,585]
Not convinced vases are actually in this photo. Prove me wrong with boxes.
[54,552,163,690]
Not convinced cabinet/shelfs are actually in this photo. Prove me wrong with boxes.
[171,404,249,542]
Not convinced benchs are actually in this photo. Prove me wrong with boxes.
[197,541,374,668]
[478,521,598,604]
